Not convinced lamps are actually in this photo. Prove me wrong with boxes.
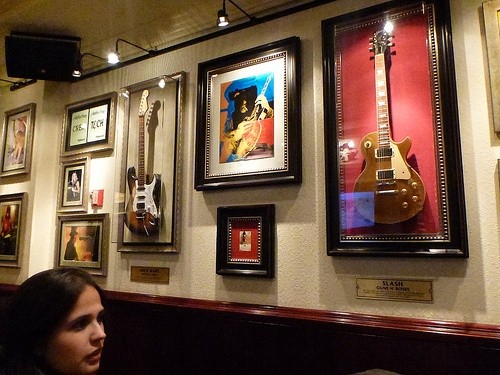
[216,0,256,27]
[73,53,107,77]
[107,38,158,64]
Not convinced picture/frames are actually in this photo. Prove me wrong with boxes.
[481,0,500,131]
[215,203,275,277]
[55,213,109,277]
[321,0,470,258]
[58,90,116,157]
[0,102,35,178]
[194,36,303,191]
[0,192,28,268]
[56,157,91,213]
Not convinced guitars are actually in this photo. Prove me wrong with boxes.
[124,88,163,236]
[350,27,425,224]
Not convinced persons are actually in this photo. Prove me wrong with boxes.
[64,227,78,260]
[220,85,274,163]
[0,267,106,375]
[0,206,14,254]
[67,173,80,201]
